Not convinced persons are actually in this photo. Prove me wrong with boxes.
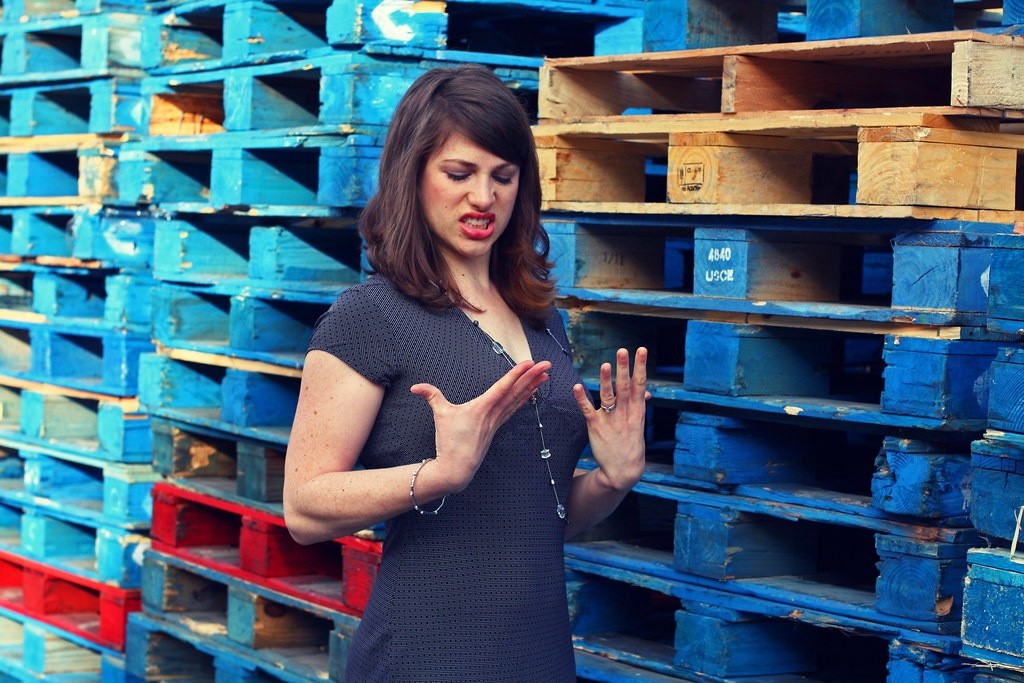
[283,66,651,683]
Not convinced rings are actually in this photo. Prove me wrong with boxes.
[601,404,616,413]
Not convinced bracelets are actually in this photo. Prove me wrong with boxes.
[410,457,446,515]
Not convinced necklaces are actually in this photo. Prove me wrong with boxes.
[474,321,565,518]
